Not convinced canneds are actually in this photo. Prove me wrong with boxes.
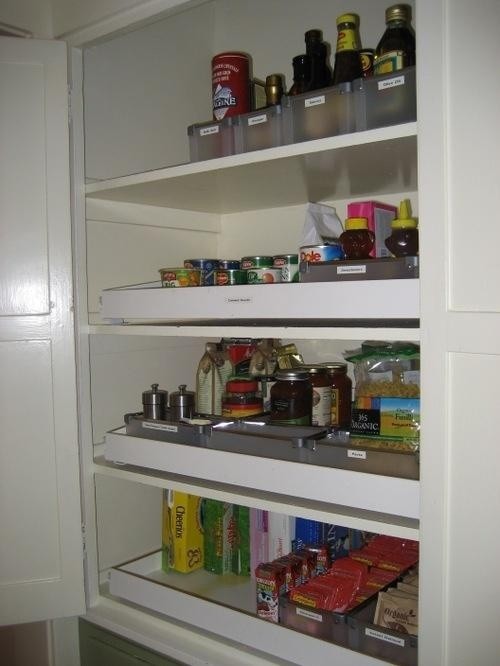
[221,362,352,431]
[211,52,254,122]
[159,253,298,288]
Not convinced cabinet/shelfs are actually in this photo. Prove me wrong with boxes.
[79,615,188,666]
[0,1,499,666]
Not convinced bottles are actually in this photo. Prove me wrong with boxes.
[142,336,388,429]
[384,200,418,257]
[212,3,414,159]
[341,217,376,259]
